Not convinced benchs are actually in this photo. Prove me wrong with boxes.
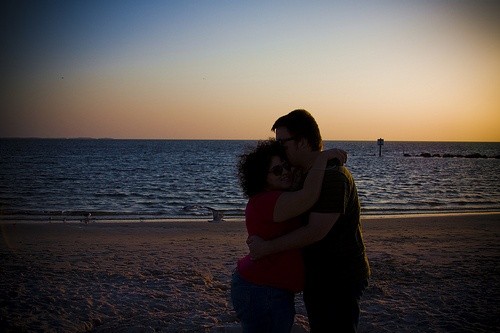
[205,206,246,222]
